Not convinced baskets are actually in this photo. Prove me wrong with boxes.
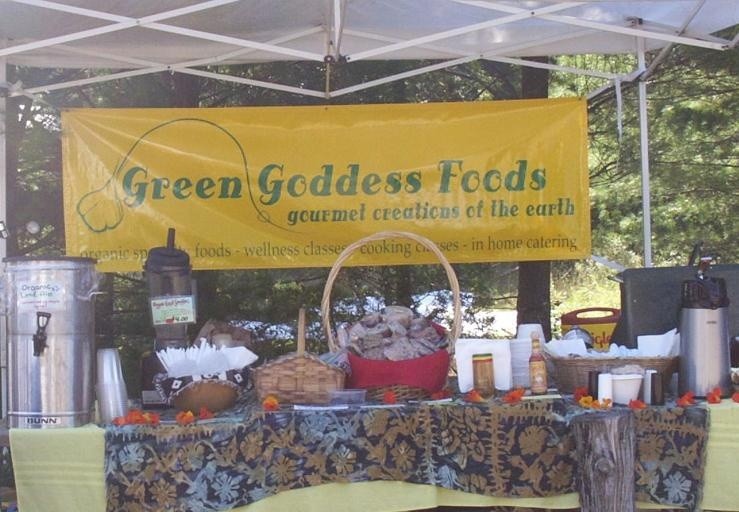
[253,307,346,406]
[545,352,680,396]
[321,230,463,403]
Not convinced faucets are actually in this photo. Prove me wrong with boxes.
[32,312,52,356]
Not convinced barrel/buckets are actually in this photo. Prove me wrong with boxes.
[0,255,98,429]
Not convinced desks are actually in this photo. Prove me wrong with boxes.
[3,378,739,512]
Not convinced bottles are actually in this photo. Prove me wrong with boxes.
[473,352,496,398]
[529,338,548,395]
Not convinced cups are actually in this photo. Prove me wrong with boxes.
[92,345,132,427]
